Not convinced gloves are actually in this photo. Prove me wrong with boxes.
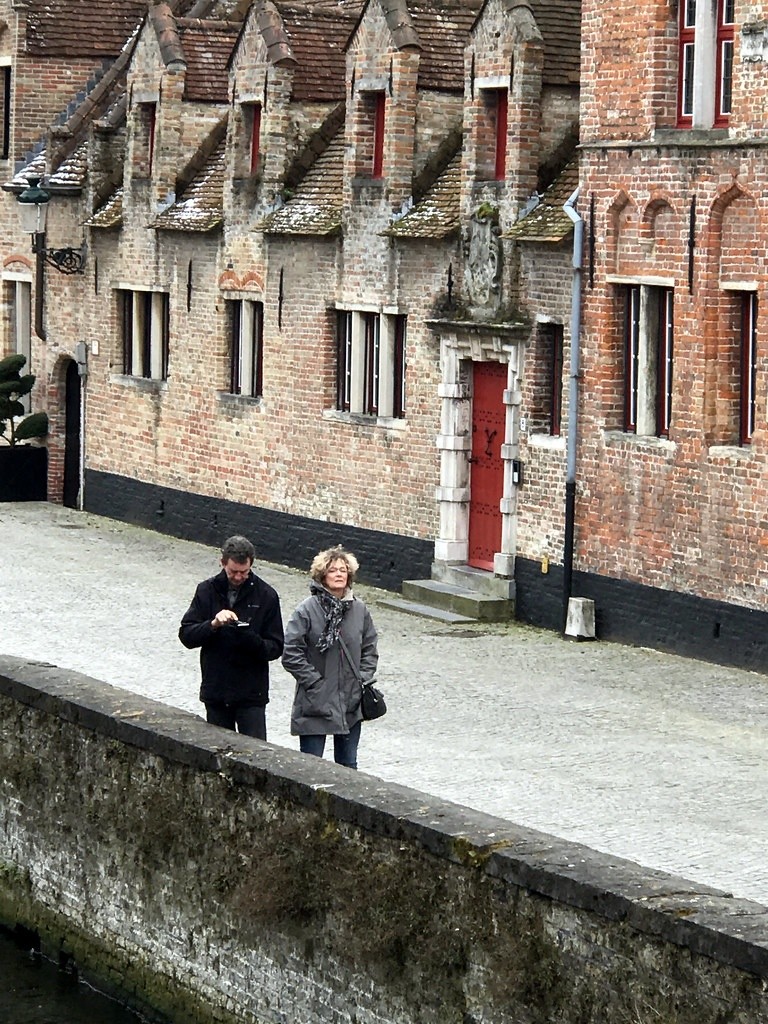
[223,617,262,650]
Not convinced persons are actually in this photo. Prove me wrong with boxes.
[282,544,377,772]
[179,534,286,742]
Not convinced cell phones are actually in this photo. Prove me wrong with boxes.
[235,620,250,627]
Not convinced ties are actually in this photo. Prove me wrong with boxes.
[229,587,237,610]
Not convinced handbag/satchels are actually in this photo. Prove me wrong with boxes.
[362,678,386,720]
